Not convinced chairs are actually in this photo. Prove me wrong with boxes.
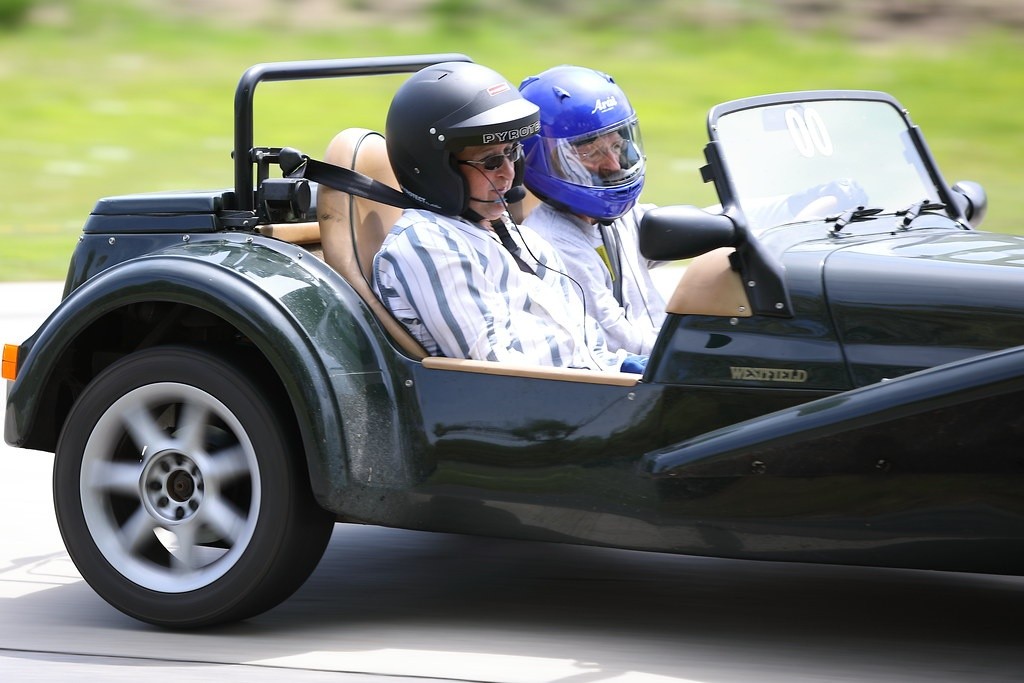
[315,128,429,362]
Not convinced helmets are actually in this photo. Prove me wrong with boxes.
[385,60,540,215]
[511,65,646,221]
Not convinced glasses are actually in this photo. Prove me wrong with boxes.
[459,143,522,170]
[556,138,628,163]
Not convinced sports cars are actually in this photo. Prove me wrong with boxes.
[2,52,1024,636]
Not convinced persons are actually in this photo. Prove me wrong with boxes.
[373,62,861,375]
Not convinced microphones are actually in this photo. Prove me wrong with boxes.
[471,186,526,203]
